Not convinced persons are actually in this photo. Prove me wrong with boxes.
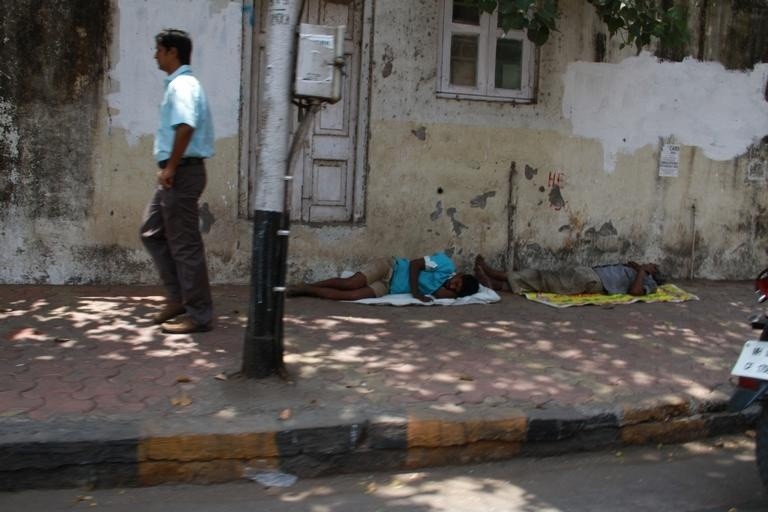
[474,252,666,296]
[285,250,479,302]
[138,29,215,334]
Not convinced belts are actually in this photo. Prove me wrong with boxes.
[159,156,204,168]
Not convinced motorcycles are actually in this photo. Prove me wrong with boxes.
[724,270,767,487]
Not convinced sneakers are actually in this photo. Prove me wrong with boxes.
[161,314,214,333]
[154,300,186,324]
